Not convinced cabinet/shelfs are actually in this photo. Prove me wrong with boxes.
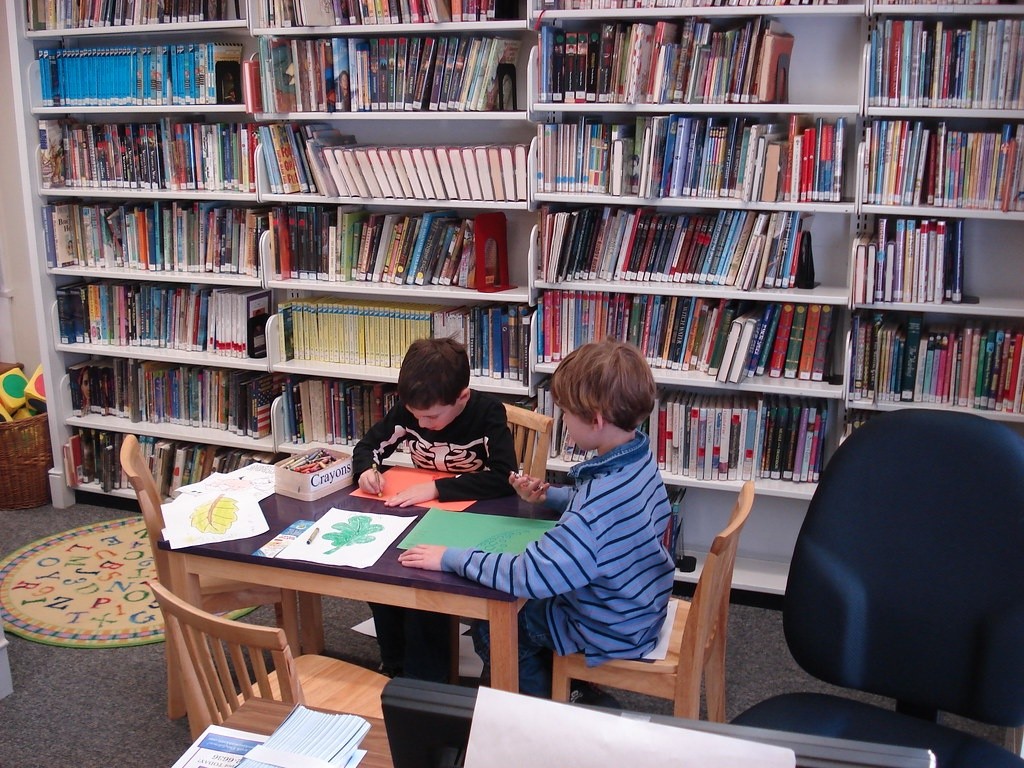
[6,0,1024,597]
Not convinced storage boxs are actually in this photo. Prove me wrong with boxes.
[274,446,354,501]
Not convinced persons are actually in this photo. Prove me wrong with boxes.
[398,340,676,707]
[352,338,518,680]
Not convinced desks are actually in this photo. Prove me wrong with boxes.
[156,483,562,694]
[219,694,394,768]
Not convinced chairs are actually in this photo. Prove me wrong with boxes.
[729,409,1024,768]
[119,435,303,721]
[145,578,392,727]
[502,401,554,484]
[552,481,756,723]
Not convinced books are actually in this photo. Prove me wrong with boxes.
[22,0,1024,568]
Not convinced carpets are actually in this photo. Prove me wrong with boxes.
[0,516,261,649]
